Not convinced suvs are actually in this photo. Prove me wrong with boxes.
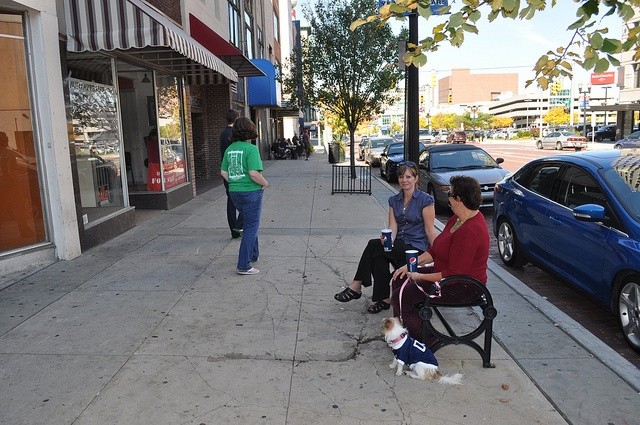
[587,124,616,140]
[419,128,435,143]
[446,130,466,143]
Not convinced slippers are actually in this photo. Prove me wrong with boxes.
[334,287,362,302]
[367,300,390,313]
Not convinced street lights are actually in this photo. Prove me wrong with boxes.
[579,82,591,136]
[471,104,478,138]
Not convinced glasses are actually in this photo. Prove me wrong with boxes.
[397,162,418,175]
[448,191,457,197]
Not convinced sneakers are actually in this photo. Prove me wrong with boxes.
[238,267,259,274]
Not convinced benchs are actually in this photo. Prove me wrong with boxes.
[391,247,496,370]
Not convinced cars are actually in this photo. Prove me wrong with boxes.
[380,141,425,181]
[436,132,448,142]
[359,137,370,159]
[364,136,393,166]
[492,150,639,353]
[535,131,589,150]
[614,130,640,148]
[391,134,404,141]
[418,144,509,209]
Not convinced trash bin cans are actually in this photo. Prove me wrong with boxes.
[328,142,340,164]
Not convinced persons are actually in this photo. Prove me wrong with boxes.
[220,116,270,275]
[333,161,437,315]
[392,175,490,354]
[219,109,245,240]
[290,129,311,151]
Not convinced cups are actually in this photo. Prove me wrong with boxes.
[405,250,419,272]
[381,229,393,252]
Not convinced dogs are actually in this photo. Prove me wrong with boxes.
[380,317,464,386]
